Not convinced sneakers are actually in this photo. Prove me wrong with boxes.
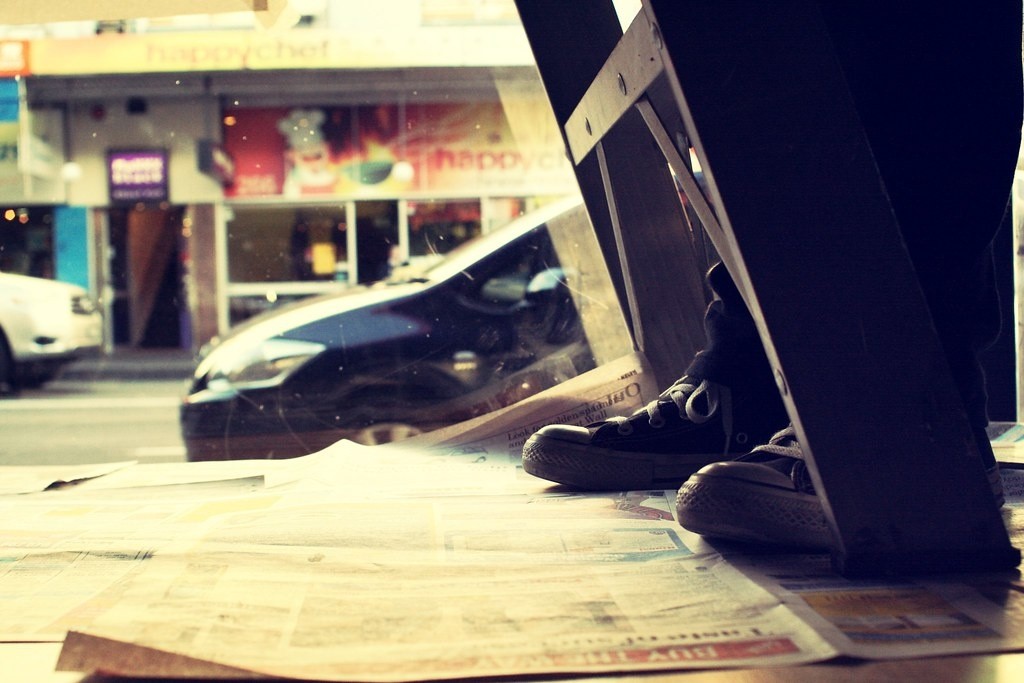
[676,420,1007,550]
[523,374,779,492]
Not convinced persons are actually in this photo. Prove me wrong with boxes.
[522,0,1024,544]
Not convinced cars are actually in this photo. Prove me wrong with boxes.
[173,169,720,467]
[0,268,107,404]
